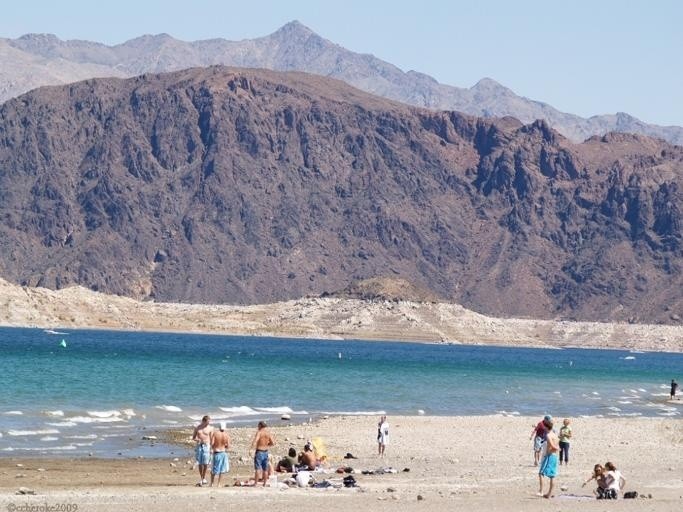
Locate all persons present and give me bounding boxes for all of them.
[274,447,298,472]
[375,415,389,459]
[297,444,316,471]
[668,378,678,399]
[556,419,573,466]
[534,418,561,499]
[250,421,274,488]
[596,461,625,502]
[257,453,274,482]
[529,414,551,467]
[581,463,609,500]
[208,420,231,487]
[307,440,313,452]
[192,415,215,487]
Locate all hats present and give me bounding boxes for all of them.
[545,416,551,420]
[219,422,227,430]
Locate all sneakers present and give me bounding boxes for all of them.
[203,479,207,483]
